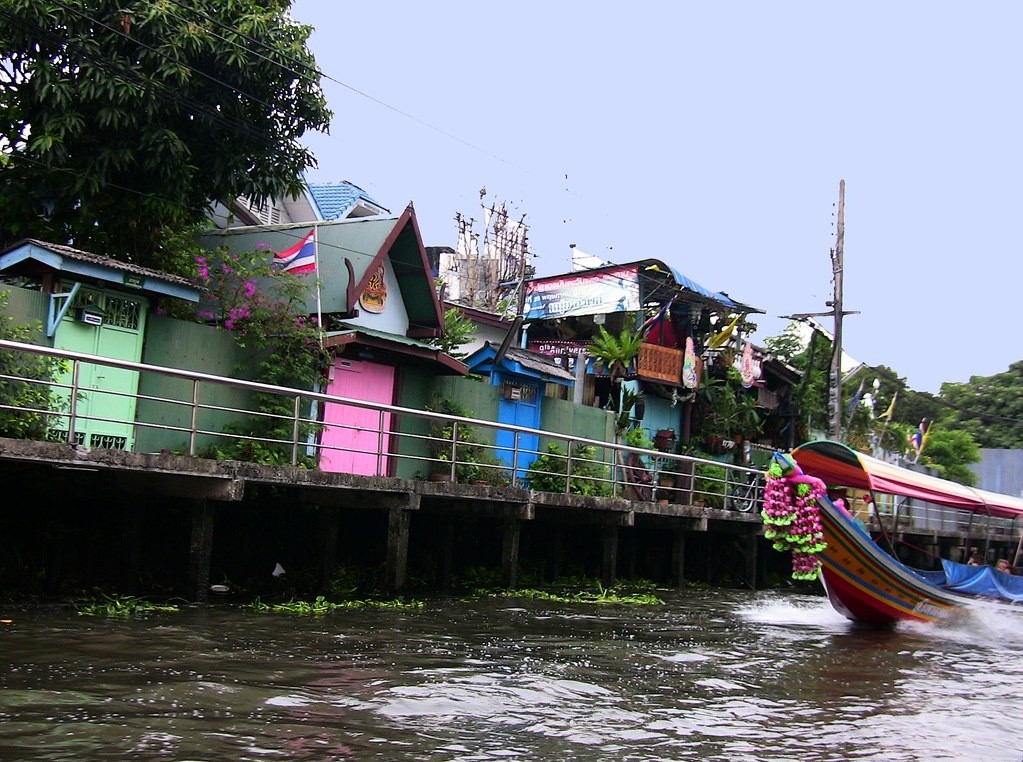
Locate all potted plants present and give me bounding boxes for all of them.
[699,370,766,455]
[473,457,511,487]
[429,426,470,482]
[660,446,694,489]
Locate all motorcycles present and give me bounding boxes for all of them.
[731,463,769,512]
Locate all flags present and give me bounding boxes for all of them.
[637,297,676,348]
[271,231,315,273]
[878,398,895,421]
[848,383,865,408]
[708,317,738,349]
[911,423,925,451]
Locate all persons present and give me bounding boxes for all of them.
[970,556,985,566]
[995,559,1010,574]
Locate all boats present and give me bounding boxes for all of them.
[761,439,1022,649]
